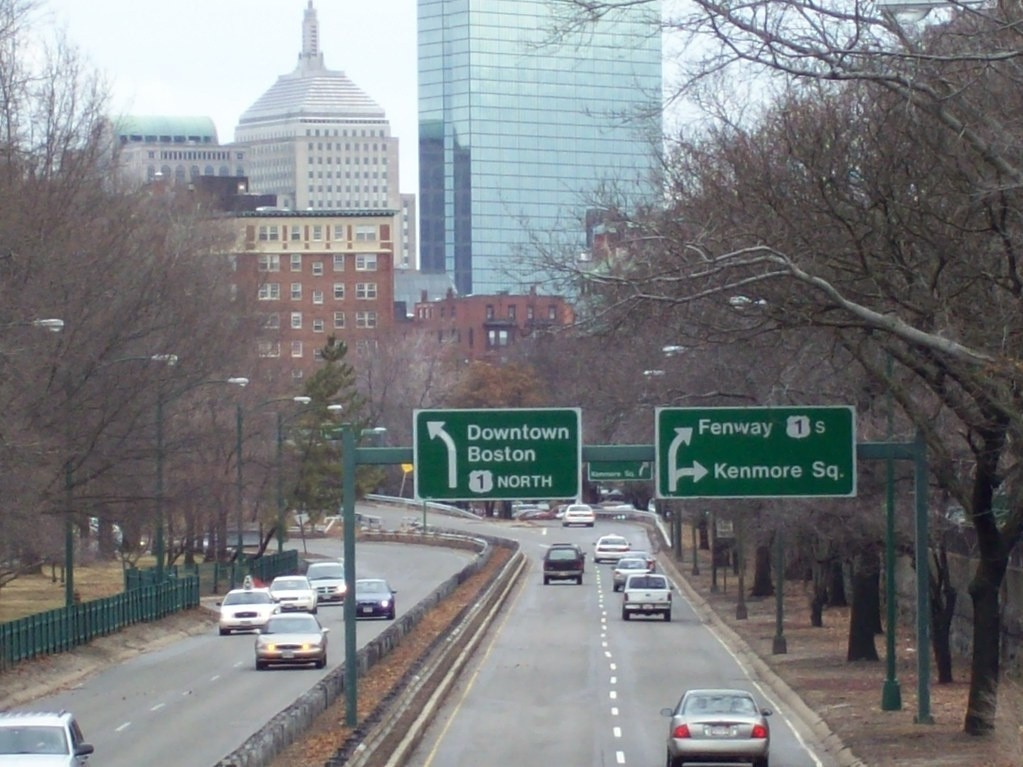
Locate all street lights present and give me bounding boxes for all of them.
[0,317,65,334]
[237,397,313,575]
[276,403,342,551]
[63,353,179,601]
[155,378,249,580]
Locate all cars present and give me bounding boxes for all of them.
[271,576,319,614]
[255,614,330,669]
[306,563,347,602]
[355,579,397,621]
[563,504,595,527]
[593,533,630,563]
[220,588,278,635]
[510,505,559,521]
[612,552,654,590]
[660,689,772,766]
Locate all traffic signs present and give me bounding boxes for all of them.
[412,408,586,502]
[588,459,655,481]
[654,403,858,500]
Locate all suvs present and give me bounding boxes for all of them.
[0,709,92,765]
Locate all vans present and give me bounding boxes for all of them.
[542,542,587,584]
[622,574,673,622]
